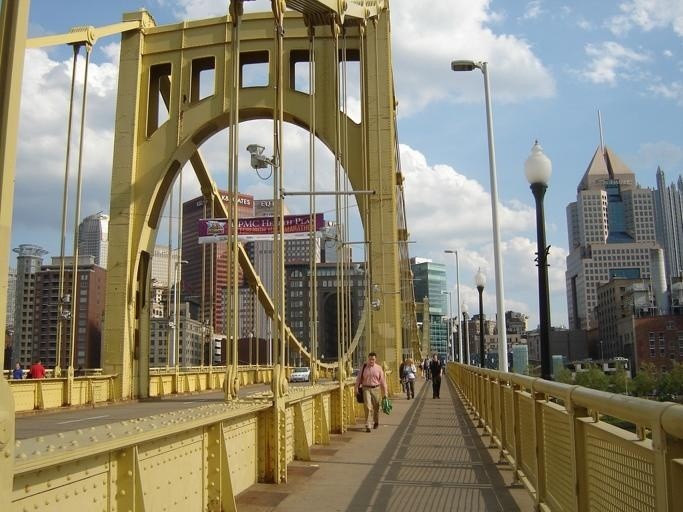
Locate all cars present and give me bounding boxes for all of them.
[336,369,360,381]
[290,367,311,382]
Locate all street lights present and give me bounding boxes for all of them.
[167,260,190,367]
[444,250,463,365]
[461,300,469,364]
[451,58,508,373]
[442,290,457,361]
[475,266,486,369]
[525,139,557,406]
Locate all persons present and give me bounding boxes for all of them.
[11,362,22,380]
[354,351,389,433]
[397,353,444,400]
[26,359,47,380]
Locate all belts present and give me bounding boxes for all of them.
[372,385,379,388]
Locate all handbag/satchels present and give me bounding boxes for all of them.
[403,374,408,384]
[356,387,363,403]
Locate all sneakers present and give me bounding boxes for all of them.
[374,423,378,429]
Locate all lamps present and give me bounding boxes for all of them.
[60,310,72,321]
[245,143,282,183]
[61,292,71,303]
